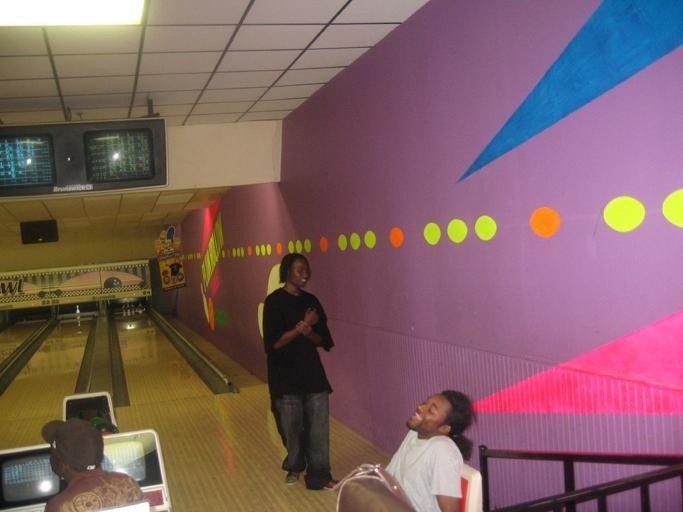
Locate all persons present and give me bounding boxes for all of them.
[40,419,149,512]
[385,390,474,512]
[261,252,340,491]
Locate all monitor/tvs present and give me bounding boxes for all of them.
[99,428,174,486]
[0,120,70,201]
[70,115,170,193]
[0,443,66,510]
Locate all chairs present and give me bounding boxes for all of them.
[459,463,483,512]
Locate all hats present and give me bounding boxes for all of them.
[42,420,104,470]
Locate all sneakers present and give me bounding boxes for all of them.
[322,479,339,491]
[287,469,299,485]
